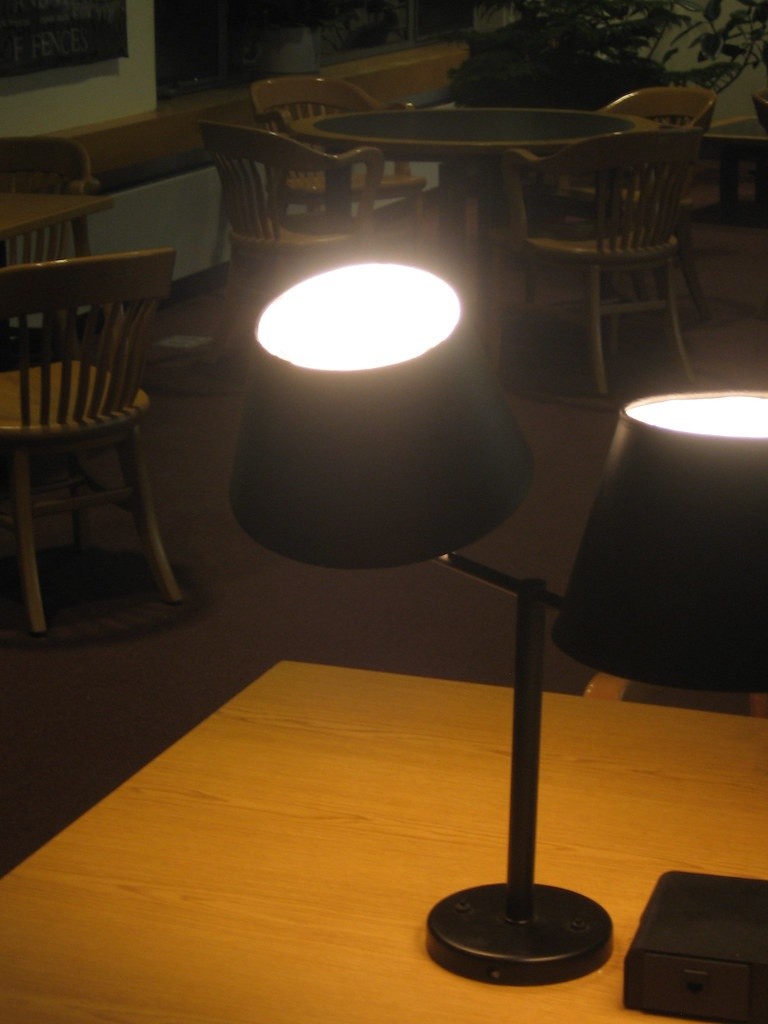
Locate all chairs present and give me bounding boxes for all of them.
[0,136,183,635]
[200,62,720,403]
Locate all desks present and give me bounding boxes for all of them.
[0,191,113,488]
[288,105,658,382]
[699,115,768,224]
[0,661,764,1024]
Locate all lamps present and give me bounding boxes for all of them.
[230,260,767,986]
